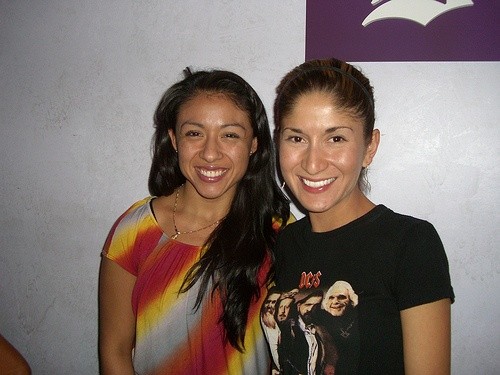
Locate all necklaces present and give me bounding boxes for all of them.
[172,185,229,240]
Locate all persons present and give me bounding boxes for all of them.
[98,66,297,375]
[259,58,454,375]
[0,335,34,375]
